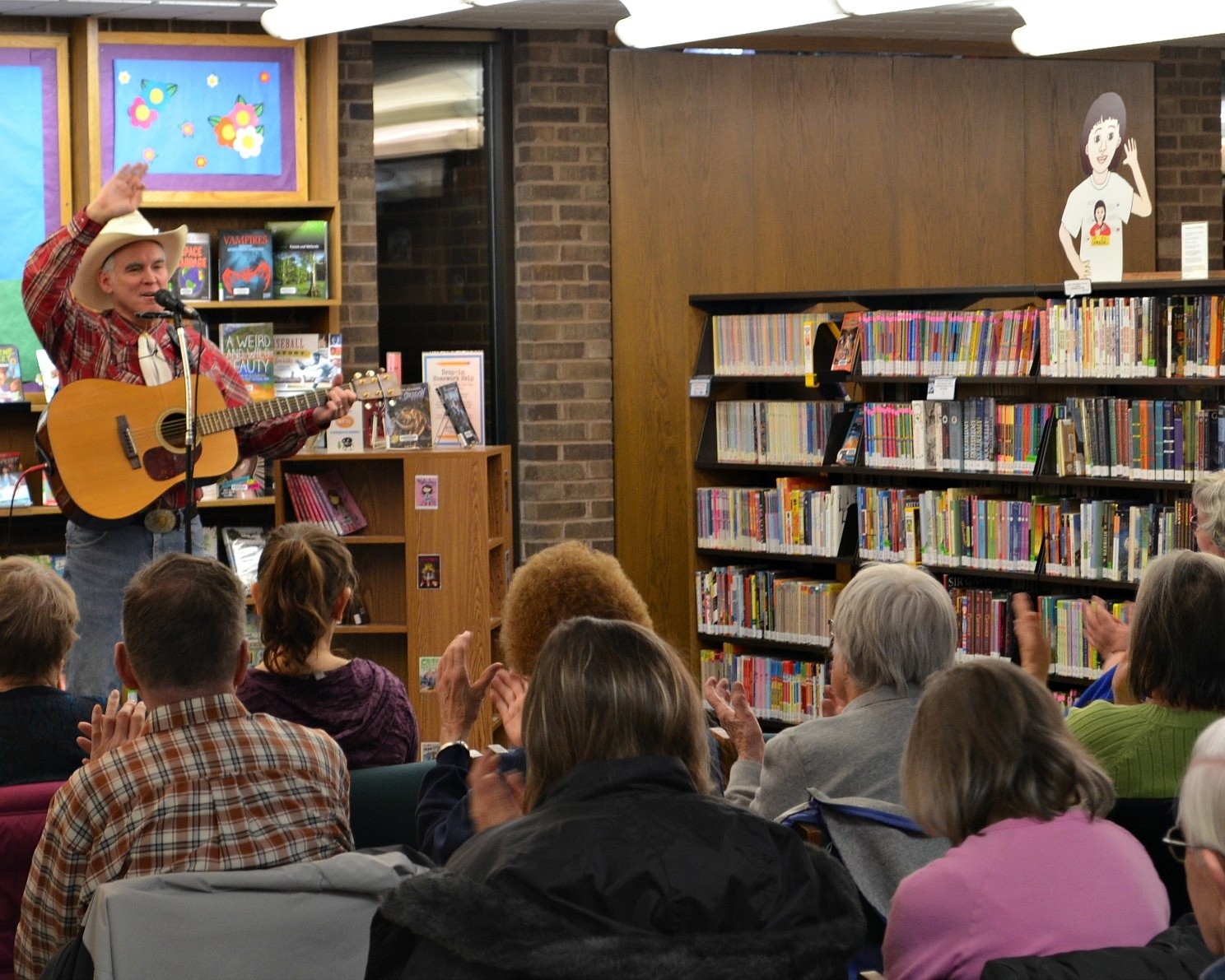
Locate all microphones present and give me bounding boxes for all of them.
[153,289,198,318]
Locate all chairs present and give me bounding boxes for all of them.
[0,774,67,980]
[1105,797,1194,928]
[348,759,436,850]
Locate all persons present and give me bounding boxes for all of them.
[365,475,1225,980]
[22,161,356,692]
[230,522,420,769]
[0,553,113,784]
[9,552,361,979]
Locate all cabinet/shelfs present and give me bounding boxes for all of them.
[688,279,1225,741]
[272,446,515,755]
[0,200,342,607]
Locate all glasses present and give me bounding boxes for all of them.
[826,618,837,635]
[1164,825,1224,862]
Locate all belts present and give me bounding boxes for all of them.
[133,500,197,533]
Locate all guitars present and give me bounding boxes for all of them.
[35,368,404,532]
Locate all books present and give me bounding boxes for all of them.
[695,295,1225,724]
[217,226,273,302]
[164,231,210,306]
[0,452,31,509]
[28,553,66,577]
[41,469,60,507]
[35,349,59,405]
[220,524,268,594]
[267,219,329,300]
[0,344,24,404]
[219,454,266,499]
[281,467,368,538]
[200,526,219,563]
[198,321,340,404]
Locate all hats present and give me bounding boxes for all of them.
[69,209,188,312]
